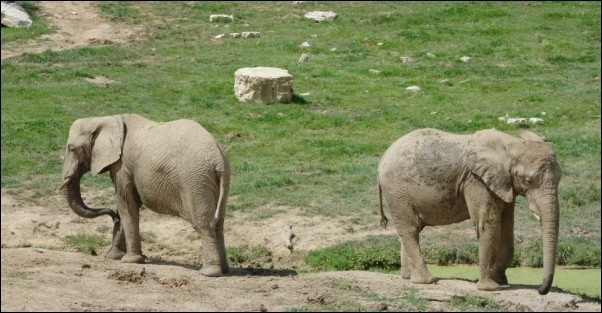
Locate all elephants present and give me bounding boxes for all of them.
[57,112,231,276]
[377,126,563,295]
